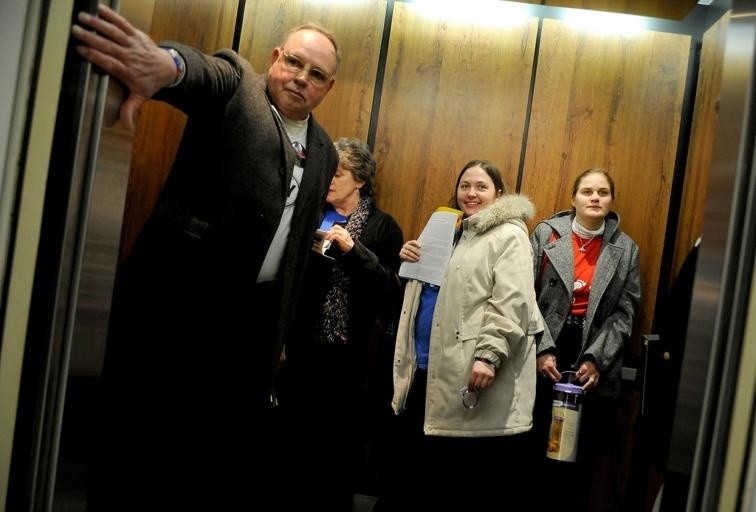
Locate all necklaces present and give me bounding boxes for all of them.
[578,227,596,253]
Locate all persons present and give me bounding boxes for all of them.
[528,168,641,510]
[388,158,545,511]
[71,3,340,511]
[292,137,404,512]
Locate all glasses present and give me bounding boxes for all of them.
[281,45,334,90]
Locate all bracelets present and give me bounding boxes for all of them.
[167,49,183,84]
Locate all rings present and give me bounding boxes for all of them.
[579,369,584,375]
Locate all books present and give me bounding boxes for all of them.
[399,207,464,287]
